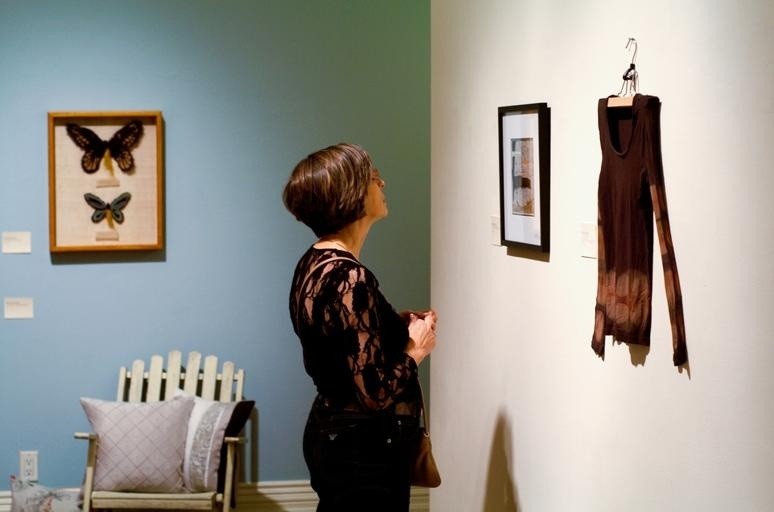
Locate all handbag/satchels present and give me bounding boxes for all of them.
[409,375,442,488]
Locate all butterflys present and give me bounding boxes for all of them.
[66,120,144,178]
[84,192,131,230]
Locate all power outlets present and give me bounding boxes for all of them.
[18,452,39,484]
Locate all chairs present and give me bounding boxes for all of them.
[73,348,248,511]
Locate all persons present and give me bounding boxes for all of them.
[281,140,442,512]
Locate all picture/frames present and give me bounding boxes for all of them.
[48,111,165,253]
[498,102,550,254]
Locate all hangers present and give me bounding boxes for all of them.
[607,37,634,109]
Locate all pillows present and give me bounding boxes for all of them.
[10,475,83,512]
[81,397,195,492]
[175,389,255,492]
